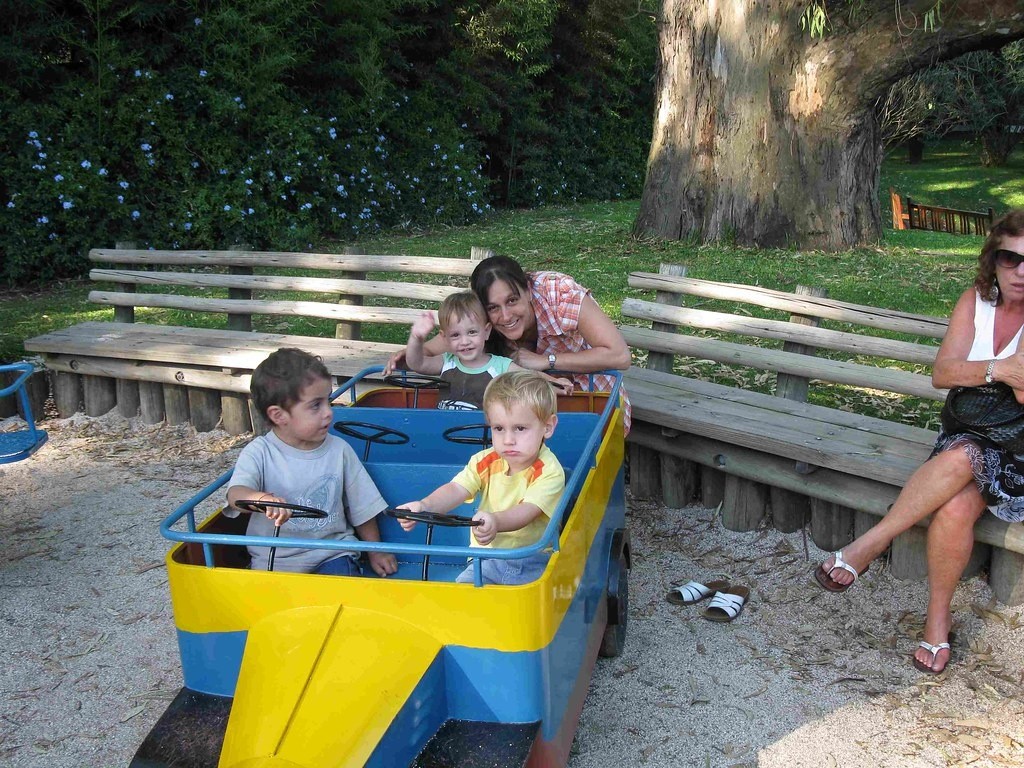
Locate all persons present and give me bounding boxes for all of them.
[395,370,566,585]
[382,256,632,438]
[406,293,573,411]
[223,347,398,578]
[813,208,1024,674]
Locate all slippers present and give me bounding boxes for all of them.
[665,579,731,606]
[702,584,750,622]
[814,549,869,592]
[913,632,956,676]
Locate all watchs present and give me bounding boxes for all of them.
[549,353,556,369]
[986,361,996,385]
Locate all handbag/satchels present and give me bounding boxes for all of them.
[941,381,1024,455]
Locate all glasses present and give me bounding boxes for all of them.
[992,248,1024,268]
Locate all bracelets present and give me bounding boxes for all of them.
[258,493,274,500]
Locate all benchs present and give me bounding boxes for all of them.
[618,264,1024,607]
[323,389,616,589]
[23,242,496,431]
